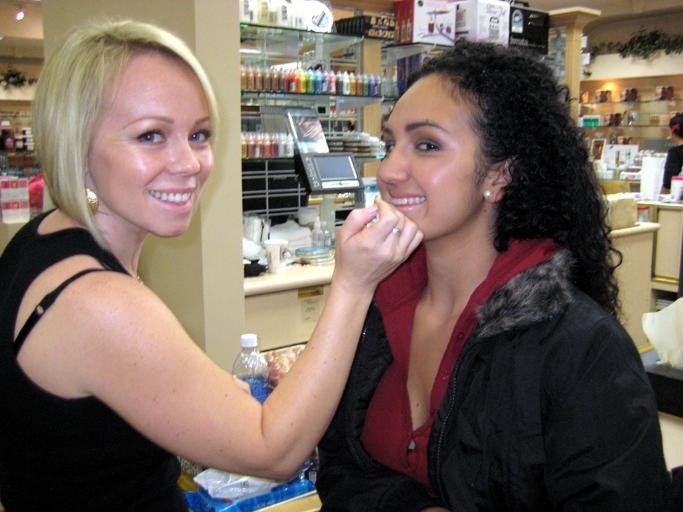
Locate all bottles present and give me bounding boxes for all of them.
[241,131,294,159]
[241,64,382,96]
[311,216,332,250]
[232,333,270,404]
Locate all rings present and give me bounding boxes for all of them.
[393,228,402,236]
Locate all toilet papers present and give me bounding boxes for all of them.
[639,157,666,200]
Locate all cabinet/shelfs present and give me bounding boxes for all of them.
[241,22,399,159]
[580,98,683,127]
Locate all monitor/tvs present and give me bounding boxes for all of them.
[300,150,364,196]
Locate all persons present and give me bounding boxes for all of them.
[316,42,668,512]
[0,20,425,512]
[660,112,683,194]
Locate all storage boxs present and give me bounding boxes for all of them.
[455,0,511,49]
[393,0,458,46]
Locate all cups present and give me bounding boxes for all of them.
[263,240,290,274]
[670,176,683,204]
